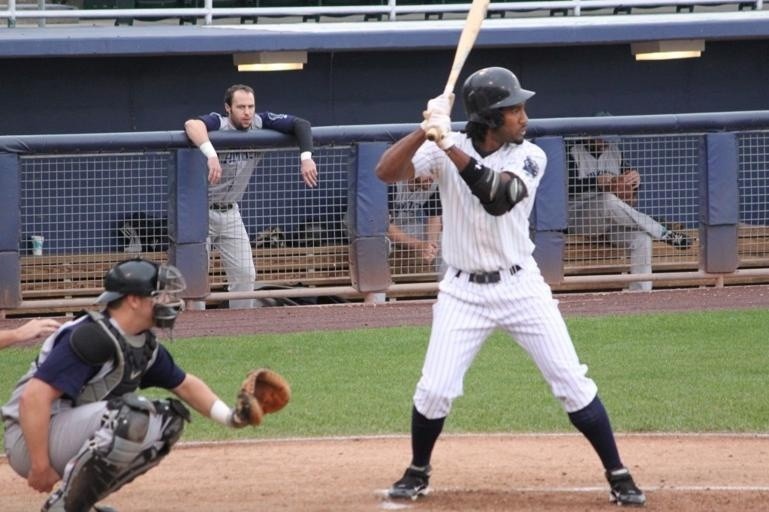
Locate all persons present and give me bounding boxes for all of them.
[0,254,253,511]
[565,108,696,292]
[183,82,320,310]
[372,63,651,507]
[0,317,65,349]
[343,162,443,303]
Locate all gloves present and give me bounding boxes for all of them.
[422,109,455,150]
[420,94,450,133]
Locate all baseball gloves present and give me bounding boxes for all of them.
[610,170,638,206]
[231,370,289,429]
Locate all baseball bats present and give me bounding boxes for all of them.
[425,0,491,139]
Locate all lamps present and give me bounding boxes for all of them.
[230,49,309,74]
[627,41,708,63]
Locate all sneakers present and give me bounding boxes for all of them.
[662,229,693,250]
[605,473,646,504]
[389,467,431,500]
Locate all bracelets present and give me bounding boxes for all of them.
[201,142,217,159]
[439,136,456,148]
[208,396,233,427]
[299,150,313,160]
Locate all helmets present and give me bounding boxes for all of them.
[93,258,187,341]
[462,66,536,127]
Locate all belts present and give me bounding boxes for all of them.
[456,264,522,285]
[210,204,232,212]
